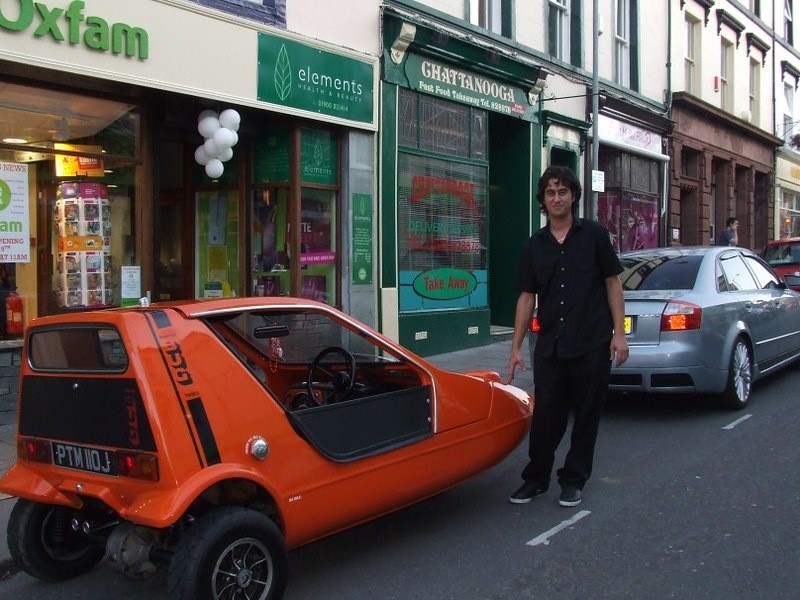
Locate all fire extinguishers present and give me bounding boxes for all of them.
[5,287,24,336]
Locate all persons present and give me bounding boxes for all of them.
[257,286,265,296]
[720,217,739,247]
[504,164,630,508]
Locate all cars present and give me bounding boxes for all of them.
[0,295,535,600]
[761,237,799,294]
[529,245,800,409]
[398,237,487,273]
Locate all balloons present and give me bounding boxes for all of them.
[194,109,241,178]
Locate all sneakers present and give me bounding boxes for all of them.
[556,475,584,507]
[509,477,554,504]
